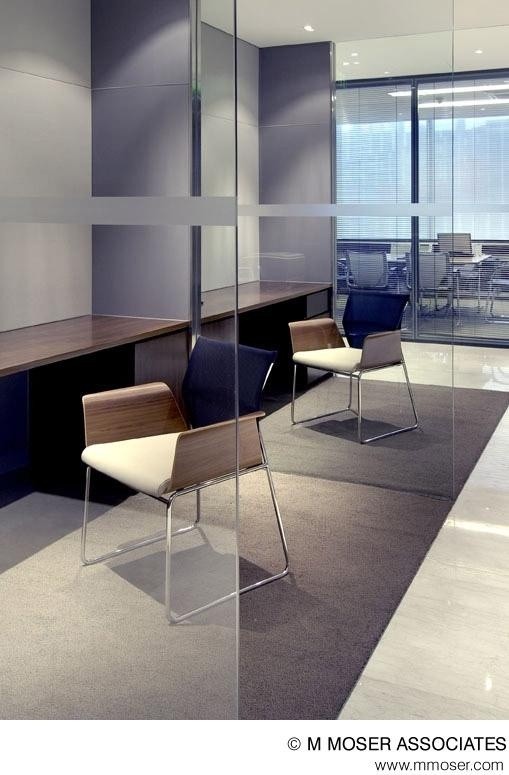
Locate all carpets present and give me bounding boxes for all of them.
[1,374,508,720]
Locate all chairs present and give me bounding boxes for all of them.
[336,231,508,329]
[285,287,421,448]
[78,334,293,627]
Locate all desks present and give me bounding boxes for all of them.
[237,277,335,394]
[0,314,194,509]
